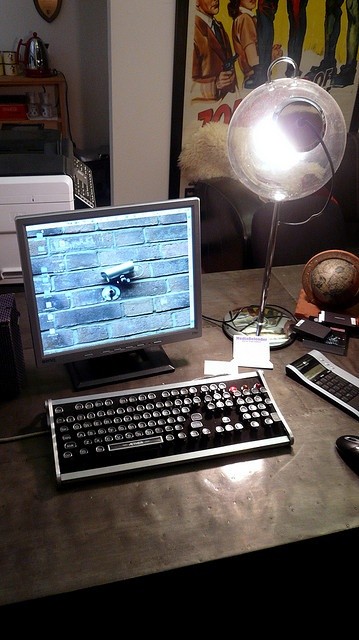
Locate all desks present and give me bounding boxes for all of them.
[0,264,359,606]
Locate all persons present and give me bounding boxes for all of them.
[245,0,308,88]
[303,0,359,88]
[190,0,236,106]
[227,0,263,88]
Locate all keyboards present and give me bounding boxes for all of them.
[42,370,288,483]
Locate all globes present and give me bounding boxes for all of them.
[302,250,359,316]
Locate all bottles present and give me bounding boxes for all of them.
[40,93,53,118]
[27,90,40,117]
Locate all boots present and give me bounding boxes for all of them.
[335,64,357,87]
[304,58,336,83]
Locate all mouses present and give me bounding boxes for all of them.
[335,435,359,479]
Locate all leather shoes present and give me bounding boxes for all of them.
[244,66,272,88]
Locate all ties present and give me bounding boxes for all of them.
[212,19,226,53]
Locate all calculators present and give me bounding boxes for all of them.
[284,348,359,421]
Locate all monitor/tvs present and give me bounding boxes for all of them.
[15,197,202,392]
[0,175,75,278]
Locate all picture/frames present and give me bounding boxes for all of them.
[167,3,359,273]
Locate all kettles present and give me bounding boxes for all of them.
[15,31,52,78]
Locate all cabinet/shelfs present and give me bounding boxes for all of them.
[1,70,66,140]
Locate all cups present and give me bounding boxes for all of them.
[4,63,19,77]
[4,50,17,64]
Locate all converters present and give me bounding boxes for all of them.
[293,318,331,341]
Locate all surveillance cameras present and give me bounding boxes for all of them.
[100,260,135,285]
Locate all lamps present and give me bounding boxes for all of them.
[223,56,347,349]
